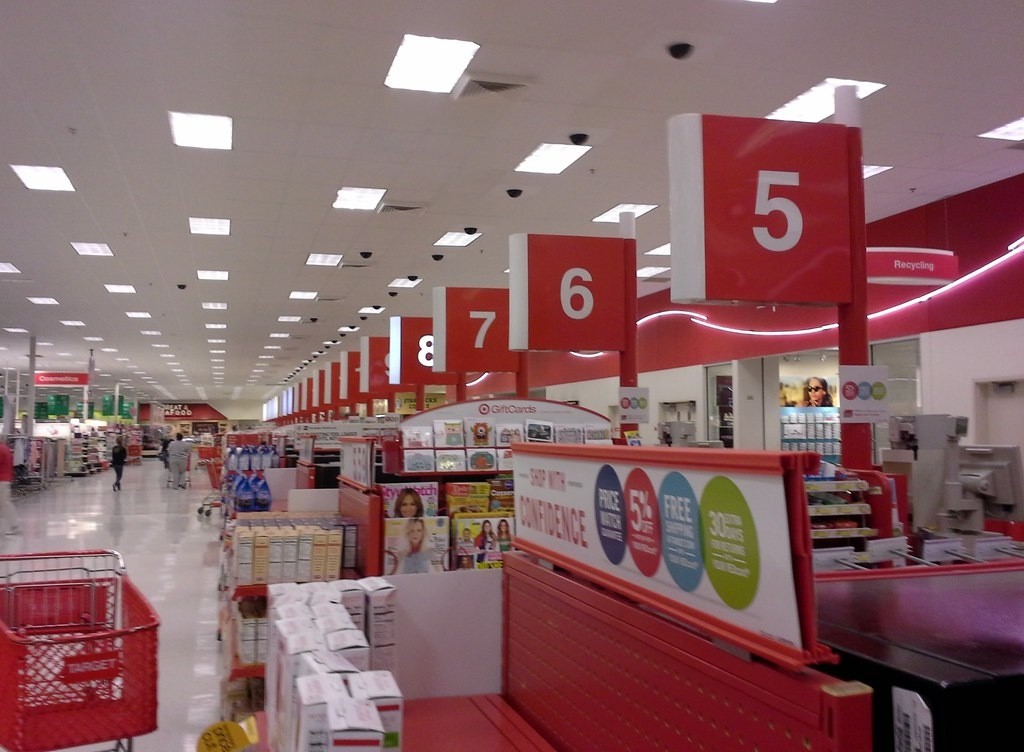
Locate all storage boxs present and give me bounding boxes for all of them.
[229,512,402,752]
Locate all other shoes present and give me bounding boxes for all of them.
[6,525,24,534]
[112,484,116,491]
[118,486,120,490]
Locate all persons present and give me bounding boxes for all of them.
[398,518,444,574]
[458,520,512,569]
[393,488,424,519]
[161,439,174,469]
[167,433,190,491]
[796,377,834,407]
[111,437,127,492]
[0,434,22,535]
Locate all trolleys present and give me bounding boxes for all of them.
[195,458,222,516]
[0,547,161,752]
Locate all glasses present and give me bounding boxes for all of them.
[808,386,823,392]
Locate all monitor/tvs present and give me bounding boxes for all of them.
[959,445,1024,522]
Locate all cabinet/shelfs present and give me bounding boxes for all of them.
[804,469,892,568]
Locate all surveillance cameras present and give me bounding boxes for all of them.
[464,228,478,234]
[570,134,590,146]
[669,44,695,59]
[507,189,522,198]
[283,252,443,384]
[177,285,186,290]
